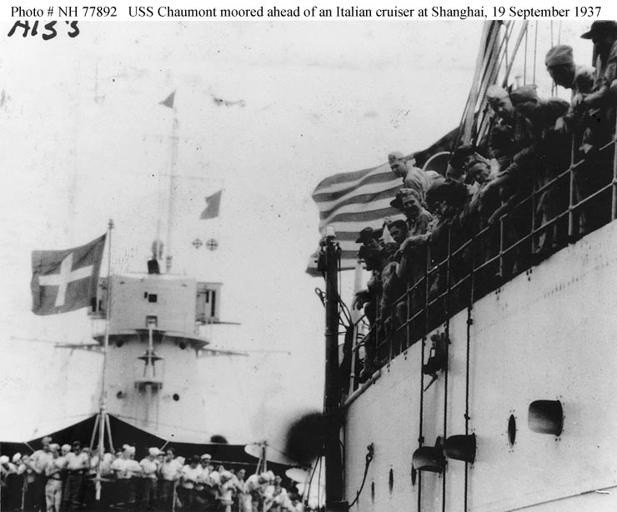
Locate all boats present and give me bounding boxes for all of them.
[342,17,617,510]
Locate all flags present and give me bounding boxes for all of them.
[200,189,222,219]
[31,231,107,315]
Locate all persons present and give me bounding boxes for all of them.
[2,441,308,511]
[355,21,615,383]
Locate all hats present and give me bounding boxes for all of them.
[2,436,312,482]
[355,19,616,246]
